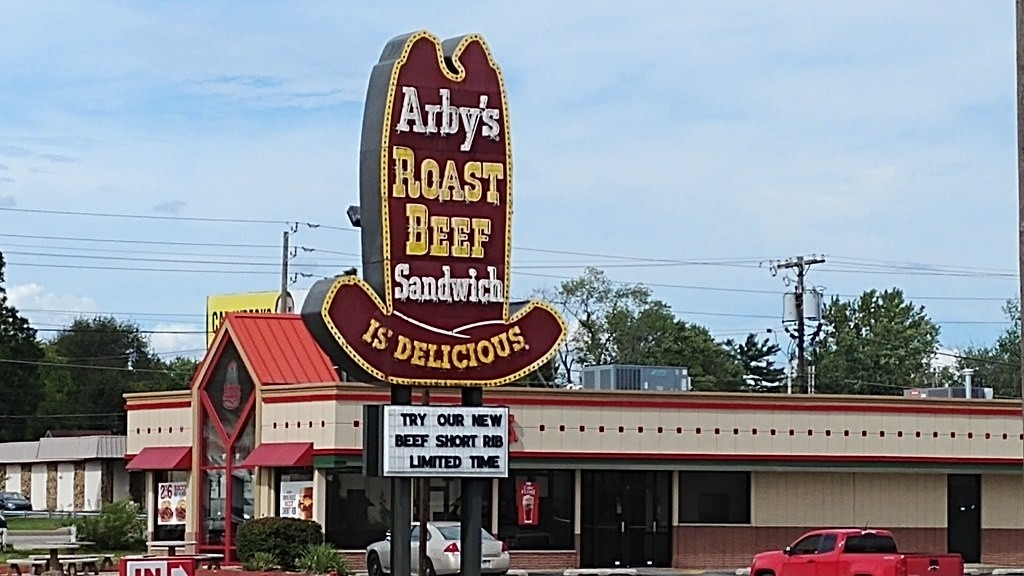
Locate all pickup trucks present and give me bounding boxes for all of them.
[748,528,965,575]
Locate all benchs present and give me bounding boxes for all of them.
[189,554,224,569]
[6,555,115,576]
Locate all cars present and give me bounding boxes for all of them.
[0,492,33,510]
[364,521,511,576]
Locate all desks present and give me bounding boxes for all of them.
[33,540,80,576]
[146,540,198,556]
[46,541,96,576]
